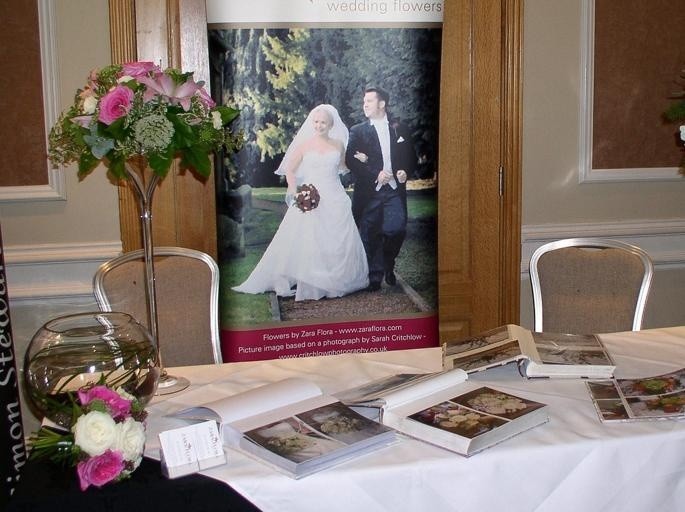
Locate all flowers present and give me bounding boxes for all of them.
[45,55,247,180]
[31,386,152,490]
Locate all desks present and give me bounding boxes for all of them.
[527,233,654,340]
[16,326,684,512]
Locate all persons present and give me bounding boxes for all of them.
[230,104,370,302]
[345,85,418,292]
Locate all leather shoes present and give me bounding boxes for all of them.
[369,272,396,290]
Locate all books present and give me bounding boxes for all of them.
[331,369,549,458]
[441,324,617,382]
[163,376,401,480]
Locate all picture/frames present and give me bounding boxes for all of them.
[576,2,685,185]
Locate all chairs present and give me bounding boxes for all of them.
[91,243,225,373]
